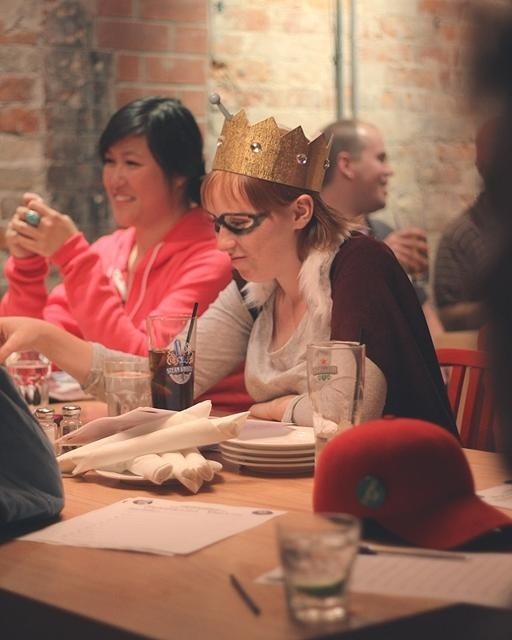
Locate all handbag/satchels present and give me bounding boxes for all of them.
[0,366,67,544]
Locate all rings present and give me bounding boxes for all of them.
[23,209,42,226]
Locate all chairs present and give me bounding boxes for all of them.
[434,348,504,453]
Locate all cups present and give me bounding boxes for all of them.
[273,512,363,623]
[8,357,54,410]
[303,340,366,468]
[102,356,152,417]
[391,203,429,286]
[145,313,197,413]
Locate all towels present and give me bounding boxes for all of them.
[56,399,251,494]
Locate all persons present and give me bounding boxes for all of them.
[433,114,510,348]
[317,116,429,307]
[0,92,256,416]
[1,167,464,448]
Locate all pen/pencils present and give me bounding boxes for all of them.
[229,573,260,616]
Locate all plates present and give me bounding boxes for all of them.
[93,461,224,487]
[219,423,315,476]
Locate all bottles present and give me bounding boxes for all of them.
[35,405,59,457]
[59,406,85,455]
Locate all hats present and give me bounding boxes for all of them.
[309,414,512,552]
